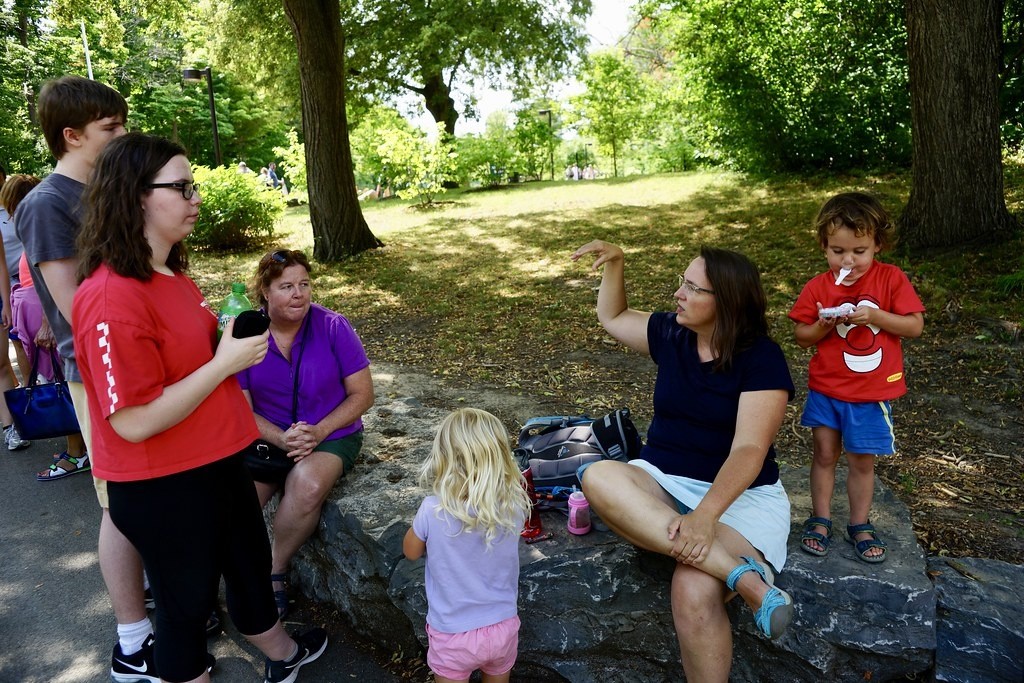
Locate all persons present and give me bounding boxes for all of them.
[786,193,926,562]
[237,160,281,188]
[565,163,596,180]
[403,408,533,683]
[15,74,224,640]
[572,239,795,683]
[0,166,89,481]
[237,248,373,620]
[71,134,329,683]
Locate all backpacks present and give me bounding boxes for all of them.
[519,406,646,507]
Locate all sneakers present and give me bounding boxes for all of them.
[264,627,328,683]
[110,634,215,683]
[144,586,158,612]
[3,427,31,450]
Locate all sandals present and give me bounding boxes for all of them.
[271,571,290,620]
[727,554,794,641]
[844,518,888,562]
[799,516,833,557]
[36,450,92,482]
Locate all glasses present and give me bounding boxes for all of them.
[678,275,715,296]
[147,181,201,199]
[259,250,306,277]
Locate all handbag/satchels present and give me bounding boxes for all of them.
[244,436,294,485]
[3,344,81,441]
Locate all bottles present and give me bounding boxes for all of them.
[217,282,254,348]
[510,448,542,537]
[567,484,592,535]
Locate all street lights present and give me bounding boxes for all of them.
[538,109,555,180]
[584,142,593,168]
[184,66,222,167]
